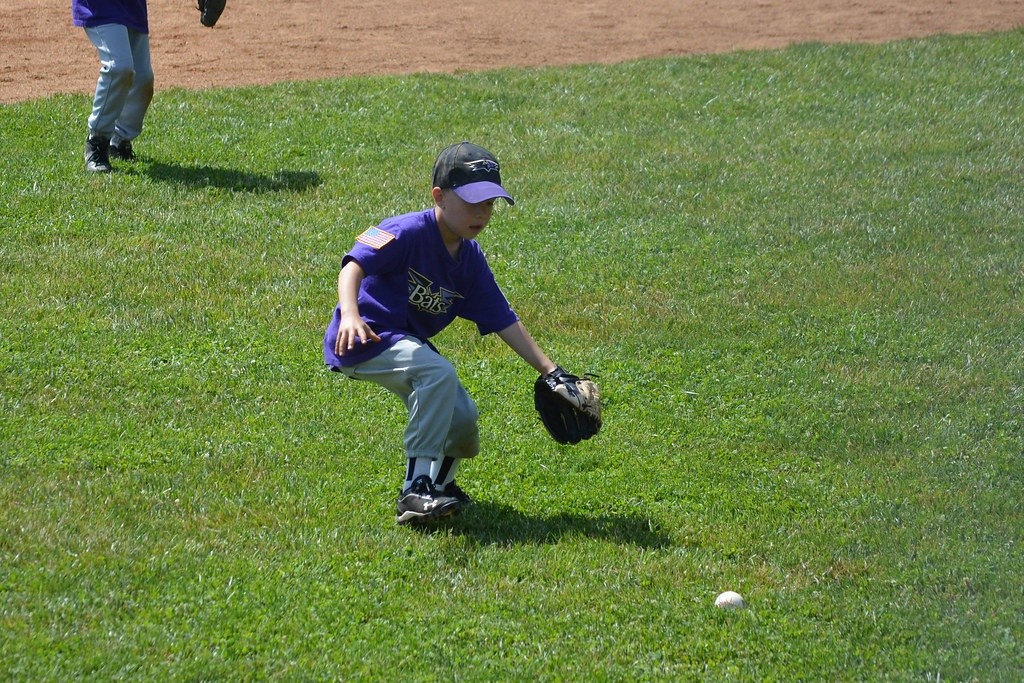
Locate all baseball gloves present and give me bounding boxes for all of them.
[534,366,605,445]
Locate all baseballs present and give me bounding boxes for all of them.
[715,589,747,611]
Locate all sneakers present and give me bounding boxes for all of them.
[394,474,459,524]
[84,134,111,173]
[108,140,136,163]
[434,478,477,507]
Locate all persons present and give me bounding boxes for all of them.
[72,0,227,170]
[324,142,603,523]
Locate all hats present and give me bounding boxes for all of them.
[432,141,515,206]
